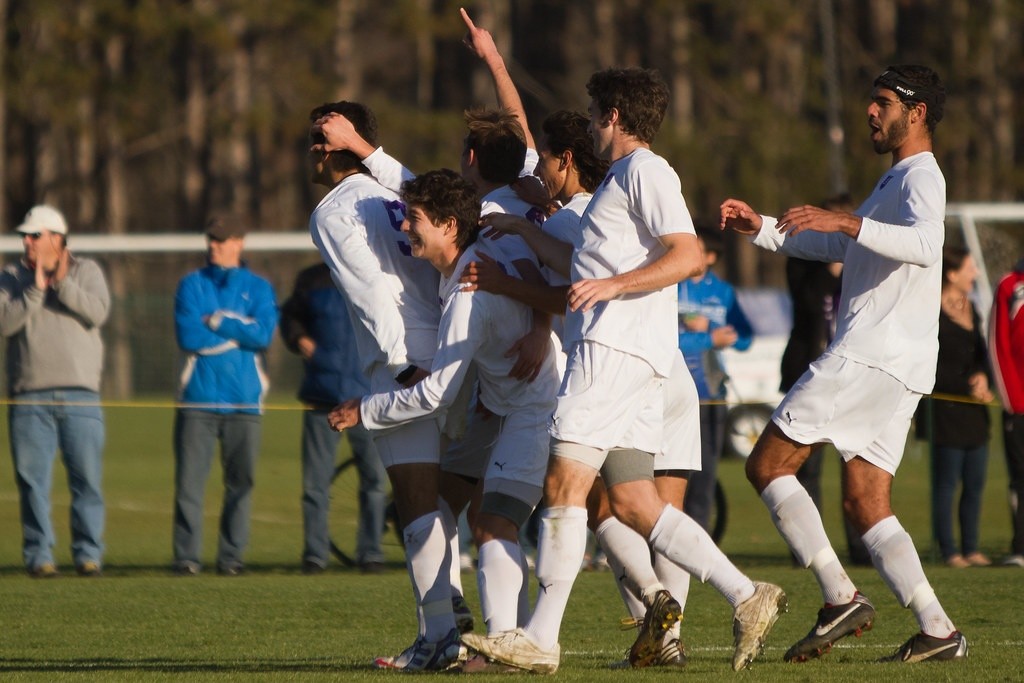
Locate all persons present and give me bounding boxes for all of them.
[779,192,878,572]
[165,210,280,577]
[1,204,113,579]
[274,260,388,576]
[677,215,758,534]
[305,6,788,670]
[927,243,998,570]
[720,65,968,664]
[988,259,1024,567]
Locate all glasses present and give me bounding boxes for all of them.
[19,232,41,240]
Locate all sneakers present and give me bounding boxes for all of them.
[373,627,467,672]
[731,582,789,672]
[462,627,560,674]
[659,639,687,666]
[629,590,684,669]
[463,652,521,673]
[784,591,876,663]
[876,629,969,665]
[450,596,475,633]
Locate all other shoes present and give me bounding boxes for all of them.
[81,563,101,576]
[36,565,59,577]
[303,561,319,574]
[1005,555,1024,567]
[219,566,236,576]
[362,563,385,574]
[179,566,195,575]
[965,554,993,567]
[946,556,969,569]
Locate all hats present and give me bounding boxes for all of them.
[205,213,247,241]
[15,205,68,238]
